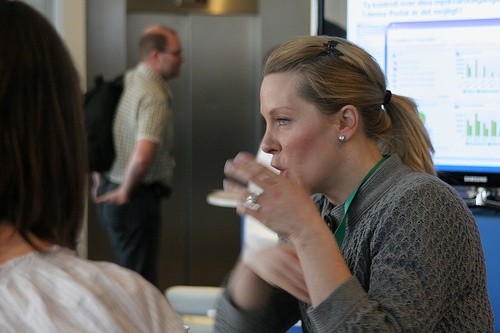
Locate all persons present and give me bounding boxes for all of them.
[213,35,495,333]
[92,25,185,287]
[0,0,190,333]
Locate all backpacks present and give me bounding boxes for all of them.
[83,71,125,177]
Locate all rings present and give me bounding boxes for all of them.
[245,192,256,204]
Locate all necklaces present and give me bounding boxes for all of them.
[319,153,390,248]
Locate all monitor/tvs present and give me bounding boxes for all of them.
[309,0,500,212]
